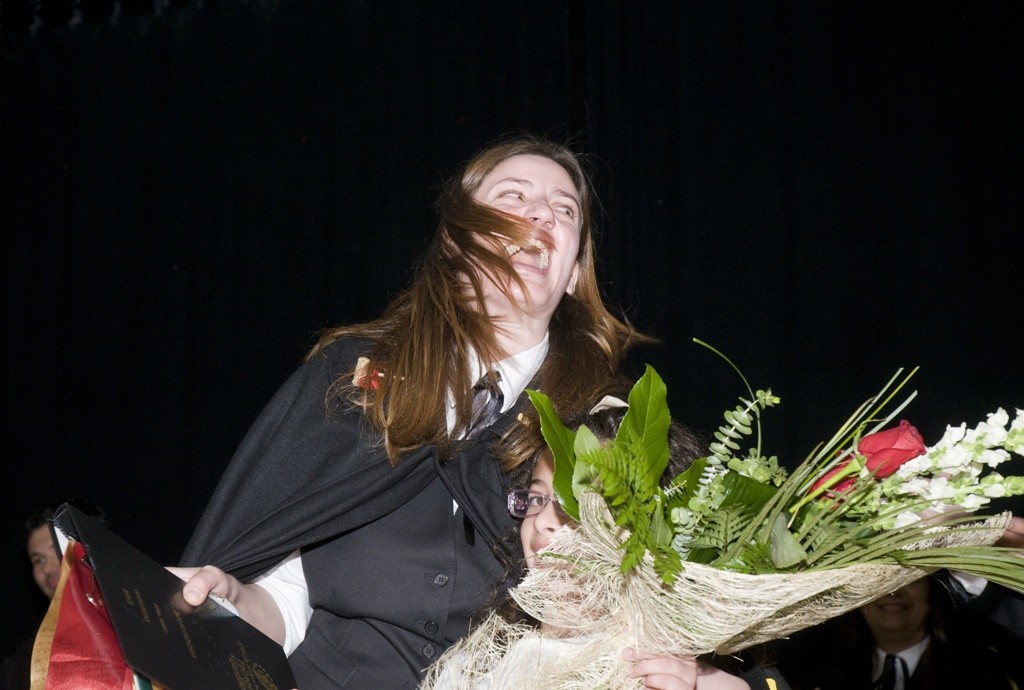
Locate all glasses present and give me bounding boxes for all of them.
[507,489,574,517]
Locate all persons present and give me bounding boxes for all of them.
[163,133,633,690]
[814,576,1013,690]
[22,500,106,603]
[493,409,749,689]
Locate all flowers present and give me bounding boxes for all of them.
[415,339,1024,690]
